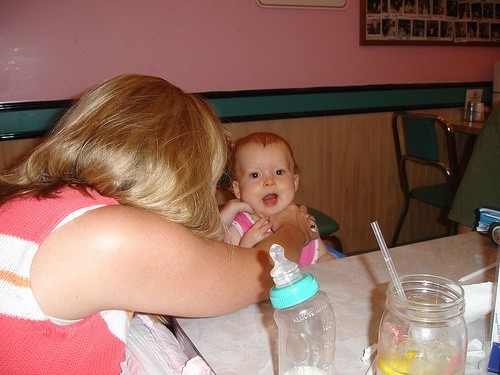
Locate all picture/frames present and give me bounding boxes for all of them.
[359,0,500,47]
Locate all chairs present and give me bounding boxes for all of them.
[388,110,459,247]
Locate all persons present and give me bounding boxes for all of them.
[367,0,500,42]
[224,131,336,268]
[0,72,320,375]
[448,97,500,237]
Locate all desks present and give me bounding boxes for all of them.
[451,114,486,134]
[174,233,500,375]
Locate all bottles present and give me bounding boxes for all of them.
[379,274,468,374]
[269,243,337,375]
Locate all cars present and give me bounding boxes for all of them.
[475,206,500,247]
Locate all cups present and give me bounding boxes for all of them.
[467,101,491,113]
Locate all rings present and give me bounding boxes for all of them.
[307,216,318,232]
